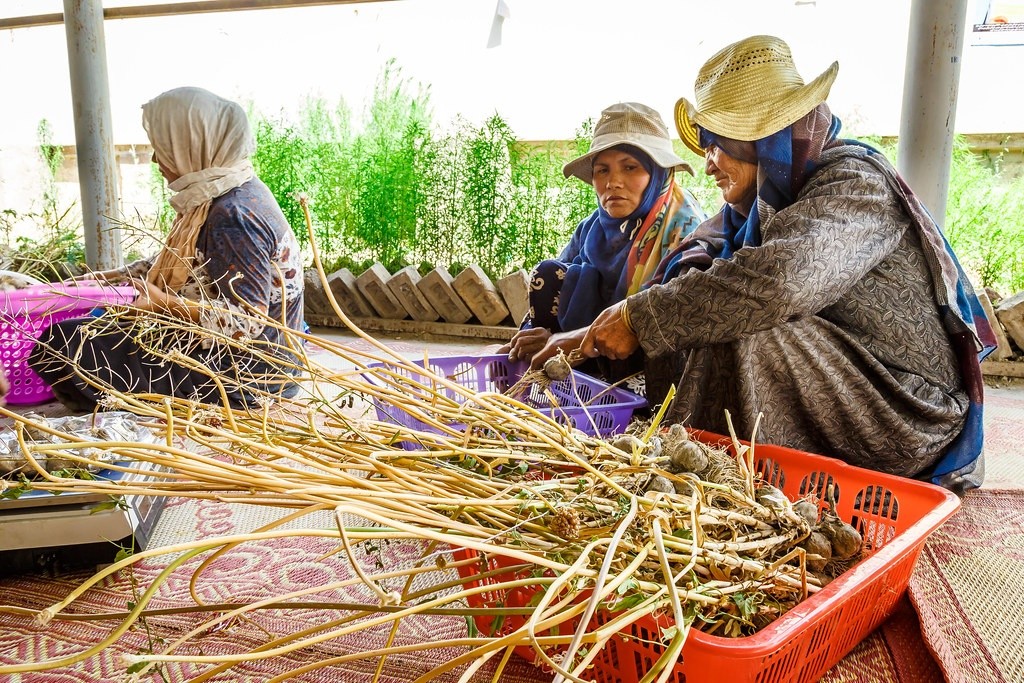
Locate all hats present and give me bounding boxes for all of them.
[676,35,839,158]
[563,102,694,182]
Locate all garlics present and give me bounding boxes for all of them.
[0,411,138,475]
[535,355,863,592]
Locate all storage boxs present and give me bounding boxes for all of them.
[357,356,647,469]
[450,426,963,683]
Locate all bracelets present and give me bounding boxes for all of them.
[621,301,637,337]
[84,272,92,279]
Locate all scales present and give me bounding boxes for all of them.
[0,433,178,574]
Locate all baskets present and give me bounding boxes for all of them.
[0,280,141,404]
[355,354,649,474]
[451,425,962,683]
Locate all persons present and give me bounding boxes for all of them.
[530,36,999,518]
[491,103,708,420]
[27,88,304,411]
